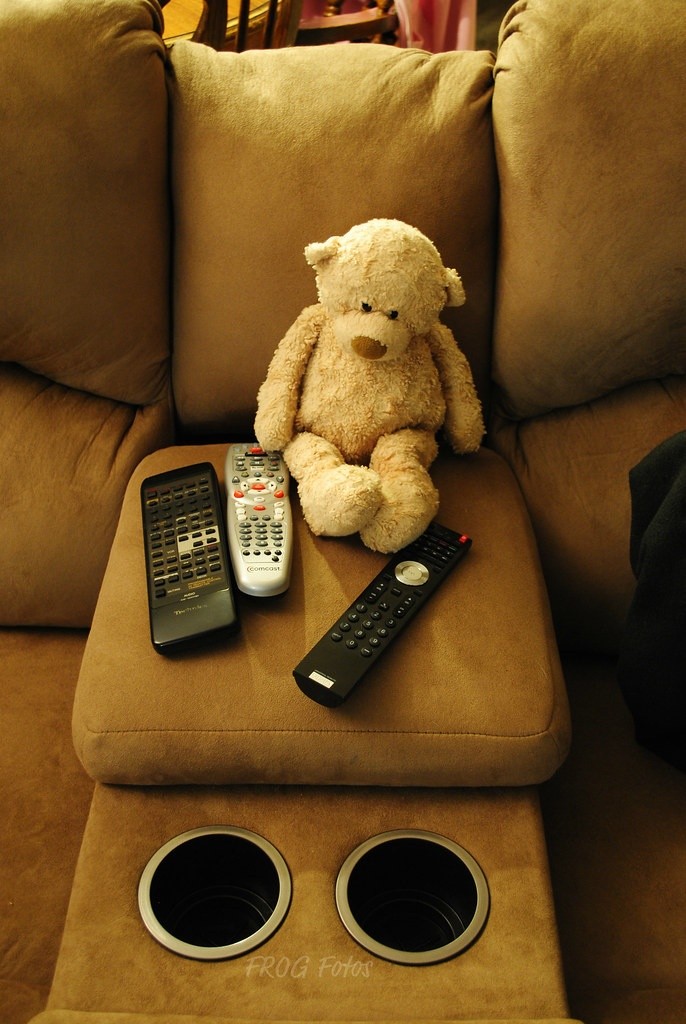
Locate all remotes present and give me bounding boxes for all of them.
[140,462,239,655]
[225,443,293,597]
[293,520,472,707]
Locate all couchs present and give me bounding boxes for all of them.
[0,1,686,1024]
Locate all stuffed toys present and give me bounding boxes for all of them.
[254,219,484,553]
